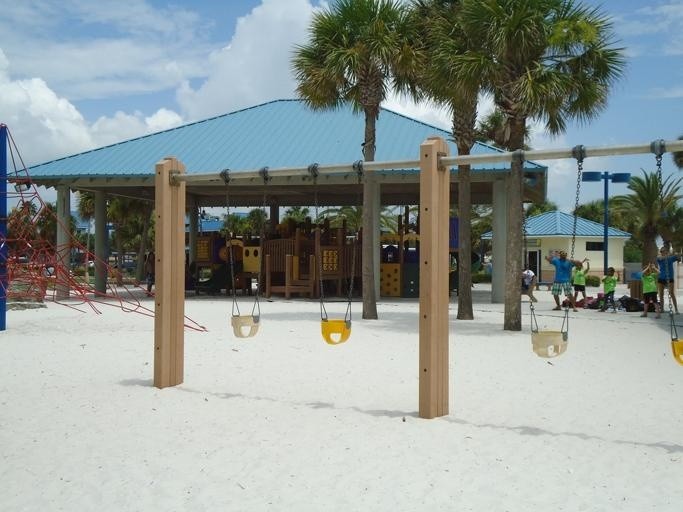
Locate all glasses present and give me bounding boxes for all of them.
[660,250,665,253]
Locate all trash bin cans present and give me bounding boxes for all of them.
[628,280,641,299]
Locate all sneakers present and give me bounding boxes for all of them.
[614,307,616,312]
[598,309,605,312]
[640,313,647,317]
[584,304,588,308]
[655,315,661,319]
[675,312,679,314]
[573,308,578,311]
[553,306,561,310]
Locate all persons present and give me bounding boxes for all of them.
[145,252,154,296]
[569,260,590,309]
[522,264,535,290]
[655,246,682,314]
[596,267,618,314]
[639,262,662,319]
[544,250,589,312]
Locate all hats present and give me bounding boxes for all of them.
[559,250,567,258]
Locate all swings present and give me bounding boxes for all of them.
[224,178,267,337]
[518,257,519,261]
[656,155,683,366]
[313,173,363,345]
[517,159,583,358]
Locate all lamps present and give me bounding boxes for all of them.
[15,182,31,192]
